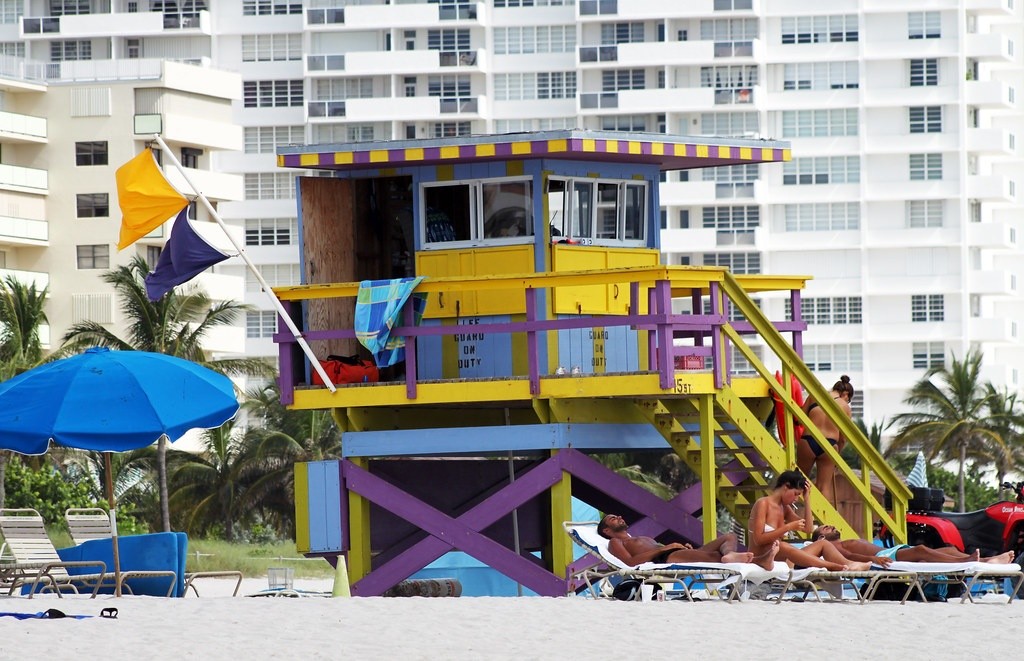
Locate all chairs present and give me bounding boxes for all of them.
[562,520,1024,604]
[0,507,70,578]
[65,507,113,546]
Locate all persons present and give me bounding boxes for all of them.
[748,467,872,572]
[812,525,1016,569]
[597,514,780,572]
[796,374,853,502]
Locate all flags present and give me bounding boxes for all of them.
[144,205,232,304]
[114,147,190,255]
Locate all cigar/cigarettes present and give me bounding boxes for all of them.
[791,501,799,510]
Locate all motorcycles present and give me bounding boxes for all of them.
[873,482,1024,601]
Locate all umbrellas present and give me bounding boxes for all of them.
[0,346,241,598]
